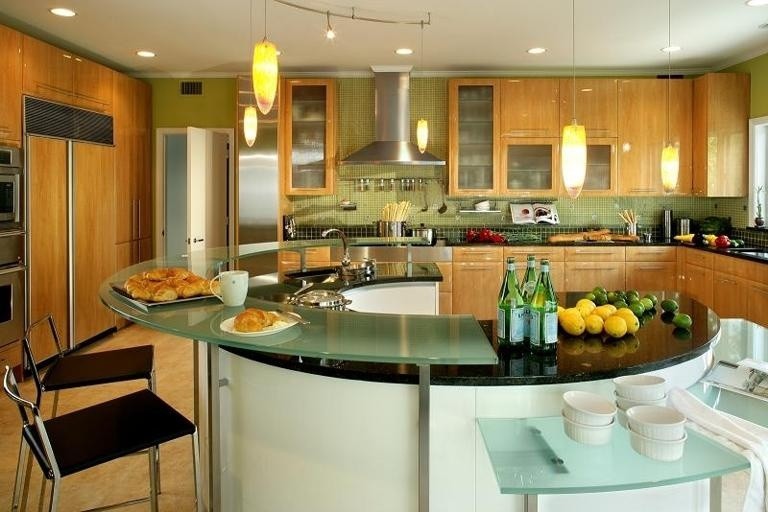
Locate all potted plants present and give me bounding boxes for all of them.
[754,186,764,226]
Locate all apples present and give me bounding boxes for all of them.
[714,235,731,249]
[466,228,507,243]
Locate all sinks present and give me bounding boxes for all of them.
[283,269,338,284]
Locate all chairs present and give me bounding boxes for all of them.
[2,360,205,512]
[17,310,163,512]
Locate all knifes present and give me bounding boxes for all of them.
[274,306,311,327]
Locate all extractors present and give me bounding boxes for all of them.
[337,66,449,166]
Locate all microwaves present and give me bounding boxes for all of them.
[0,147,21,226]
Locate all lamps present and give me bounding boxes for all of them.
[414,19,430,155]
[324,10,335,39]
[556,1,590,200]
[240,73,259,147]
[249,1,282,117]
[657,1,683,192]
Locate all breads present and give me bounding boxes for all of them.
[125,266,220,302]
[548,229,639,242]
[234,308,291,333]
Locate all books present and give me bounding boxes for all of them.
[700,356,768,404]
[510,202,560,225]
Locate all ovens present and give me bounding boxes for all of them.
[0,227,26,349]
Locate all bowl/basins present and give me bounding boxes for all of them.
[563,374,689,461]
[473,199,490,211]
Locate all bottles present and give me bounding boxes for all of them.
[496,354,558,378]
[496,253,558,351]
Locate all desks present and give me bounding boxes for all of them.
[473,318,768,512]
[96,234,500,512]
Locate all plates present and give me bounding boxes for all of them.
[233,325,303,346]
[219,310,303,338]
[108,280,219,306]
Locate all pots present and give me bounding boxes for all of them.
[297,290,350,312]
[373,220,407,246]
[410,222,437,247]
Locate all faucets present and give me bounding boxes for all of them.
[322,227,350,265]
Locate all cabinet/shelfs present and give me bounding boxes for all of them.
[678,244,714,309]
[503,245,565,295]
[447,76,500,198]
[715,253,768,329]
[617,78,692,197]
[279,75,338,196]
[23,36,113,112]
[112,236,152,331]
[566,244,625,293]
[500,78,616,199]
[690,72,748,198]
[278,245,330,285]
[115,70,153,245]
[451,248,502,321]
[0,23,23,146]
[625,245,677,293]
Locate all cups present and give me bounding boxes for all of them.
[624,222,638,236]
[210,269,249,308]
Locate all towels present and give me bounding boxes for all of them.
[668,377,768,512]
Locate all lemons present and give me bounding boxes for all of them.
[672,328,692,341]
[661,311,675,324]
[730,239,745,248]
[556,287,658,338]
[661,299,679,312]
[671,314,694,329]
[560,334,640,358]
